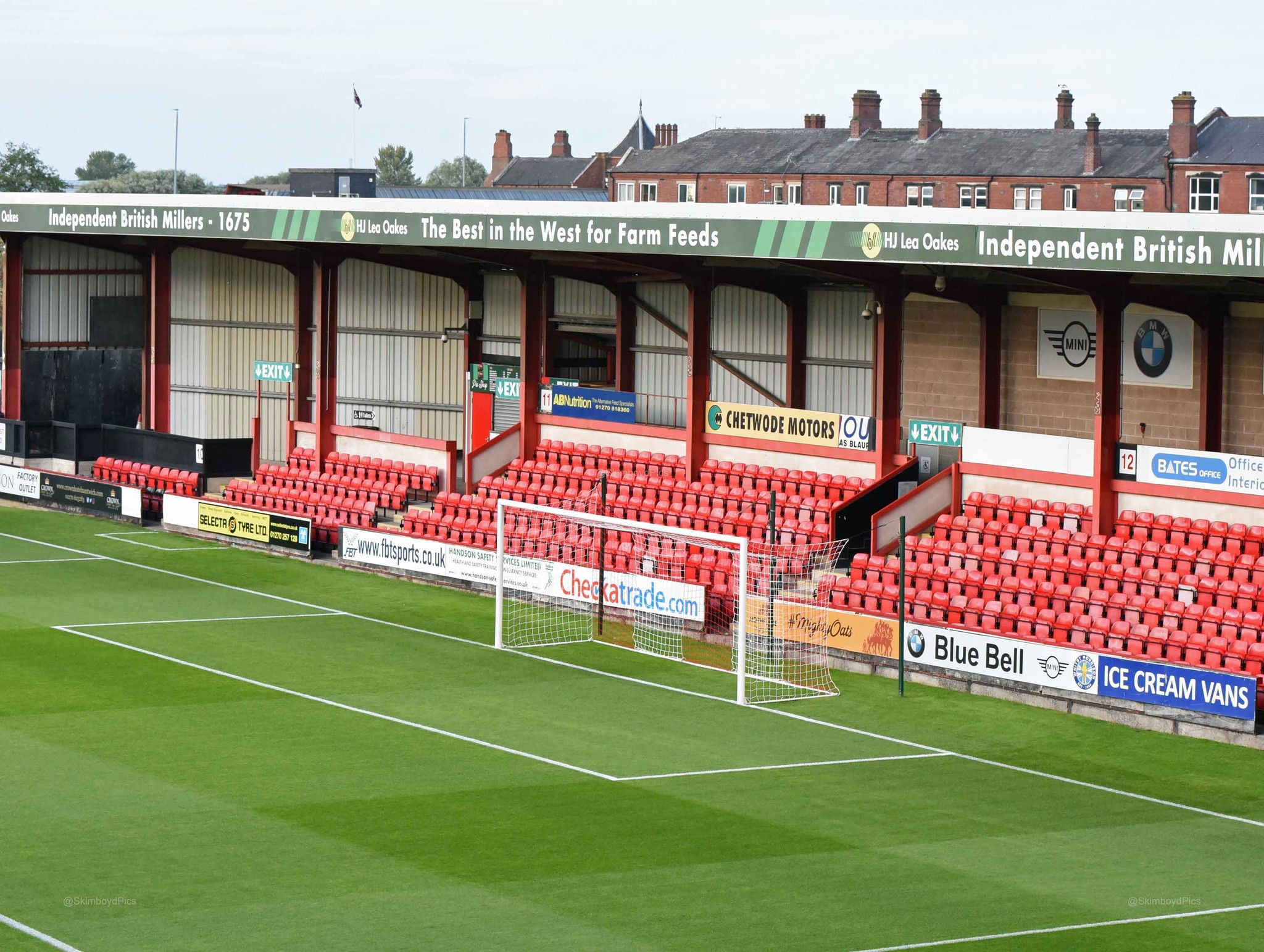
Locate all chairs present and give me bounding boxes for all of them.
[91,456,200,521]
[225,436,1264,713]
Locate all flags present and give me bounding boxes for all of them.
[354,88,362,108]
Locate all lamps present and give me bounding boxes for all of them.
[934,275,946,293]
[860,300,883,321]
[441,318,468,343]
